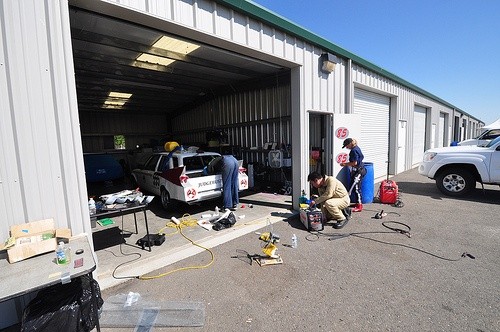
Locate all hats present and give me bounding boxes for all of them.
[341,138,353,148]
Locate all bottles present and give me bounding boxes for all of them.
[171,215,180,225]
[55,241,71,265]
[75,249,84,268]
[201,214,211,218]
[215,206,219,216]
[180,144,185,155]
[292,234,297,248]
[88,196,96,216]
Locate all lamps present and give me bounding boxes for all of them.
[320,52,337,75]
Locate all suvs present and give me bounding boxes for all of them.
[417,135,500,199]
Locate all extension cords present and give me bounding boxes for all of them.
[171,217,180,225]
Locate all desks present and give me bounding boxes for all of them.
[90,198,153,252]
[0,232,102,332]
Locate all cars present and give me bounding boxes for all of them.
[129,144,249,212]
[449,126,500,148]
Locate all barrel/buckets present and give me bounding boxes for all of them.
[348,162,374,203]
[380,180,398,204]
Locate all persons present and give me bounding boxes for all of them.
[202,155,239,213]
[307,170,352,229]
[340,138,367,212]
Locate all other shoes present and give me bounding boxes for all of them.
[352,204,362,212]
[334,219,348,229]
[229,205,238,210]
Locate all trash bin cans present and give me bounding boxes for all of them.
[19,274,104,332]
[346,161,375,204]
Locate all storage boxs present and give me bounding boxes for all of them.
[0,218,72,264]
